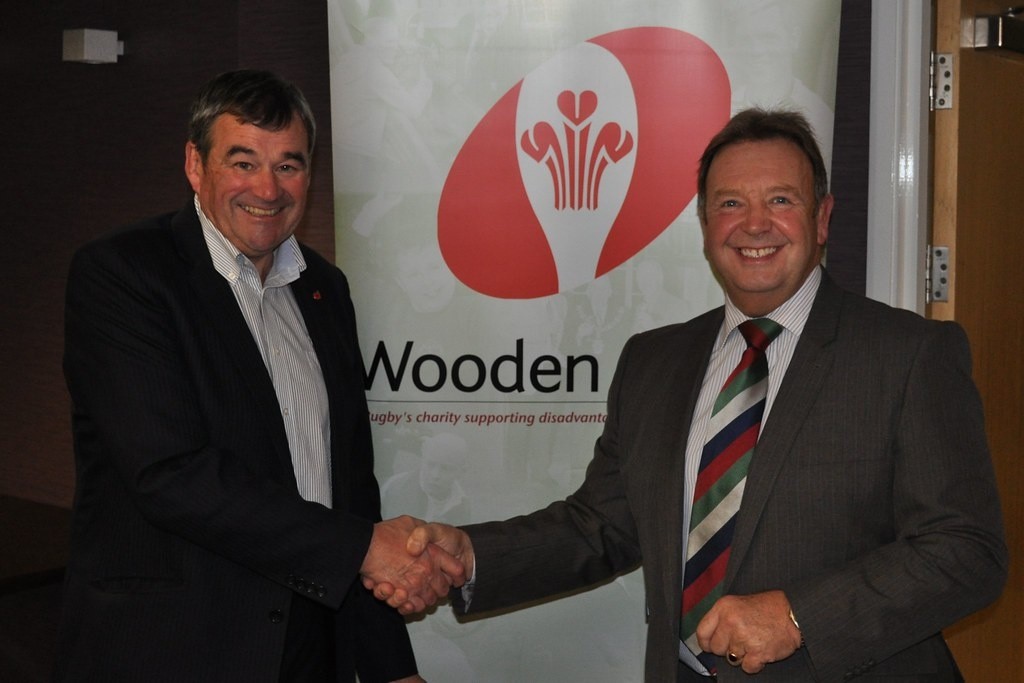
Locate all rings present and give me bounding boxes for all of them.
[729,649,743,662]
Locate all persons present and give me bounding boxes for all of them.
[363,108,1010,683]
[62,69,467,682]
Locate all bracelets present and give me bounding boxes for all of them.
[789,607,805,656]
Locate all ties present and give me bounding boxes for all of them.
[679,318,785,675]
